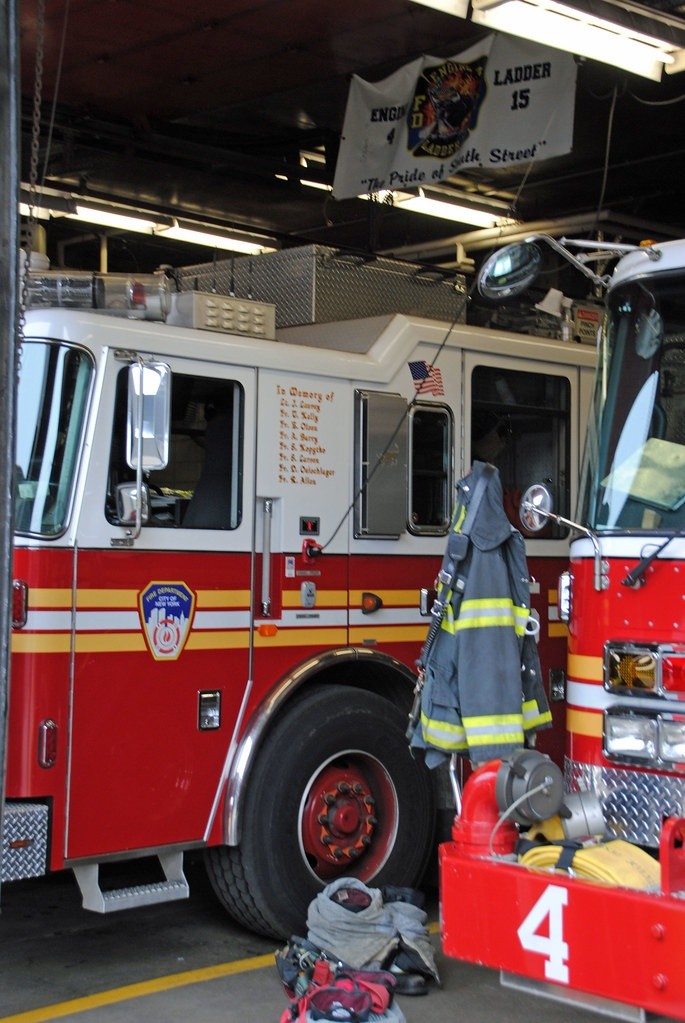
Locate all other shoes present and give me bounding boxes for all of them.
[390,970,429,995]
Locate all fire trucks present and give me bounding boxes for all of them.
[0,238,604,945]
[427,231,685,1023]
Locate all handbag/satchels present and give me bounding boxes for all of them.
[275,935,396,1014]
[281,972,371,1023]
